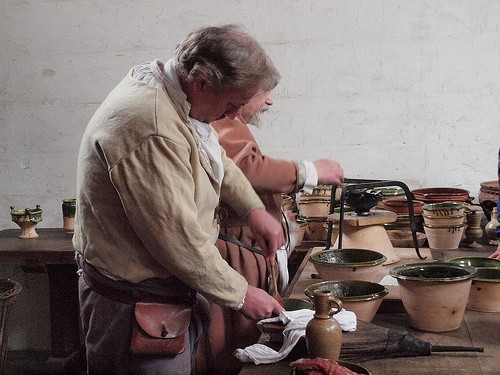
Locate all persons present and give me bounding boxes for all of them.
[195,56,344,375]
[72,23,286,375]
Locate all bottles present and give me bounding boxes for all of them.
[485,207,500,246]
[305,289,342,360]
[479,181,500,222]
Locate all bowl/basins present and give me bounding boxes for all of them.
[444,256,500,313]
[388,262,478,331]
[262,297,314,327]
[308,248,388,283]
[280,181,471,250]
[303,280,390,323]
[290,360,374,375]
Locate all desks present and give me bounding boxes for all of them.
[236,245,500,375]
[0,228,79,375]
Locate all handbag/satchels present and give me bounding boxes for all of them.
[129,302,190,357]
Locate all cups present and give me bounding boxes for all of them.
[61,199,76,234]
